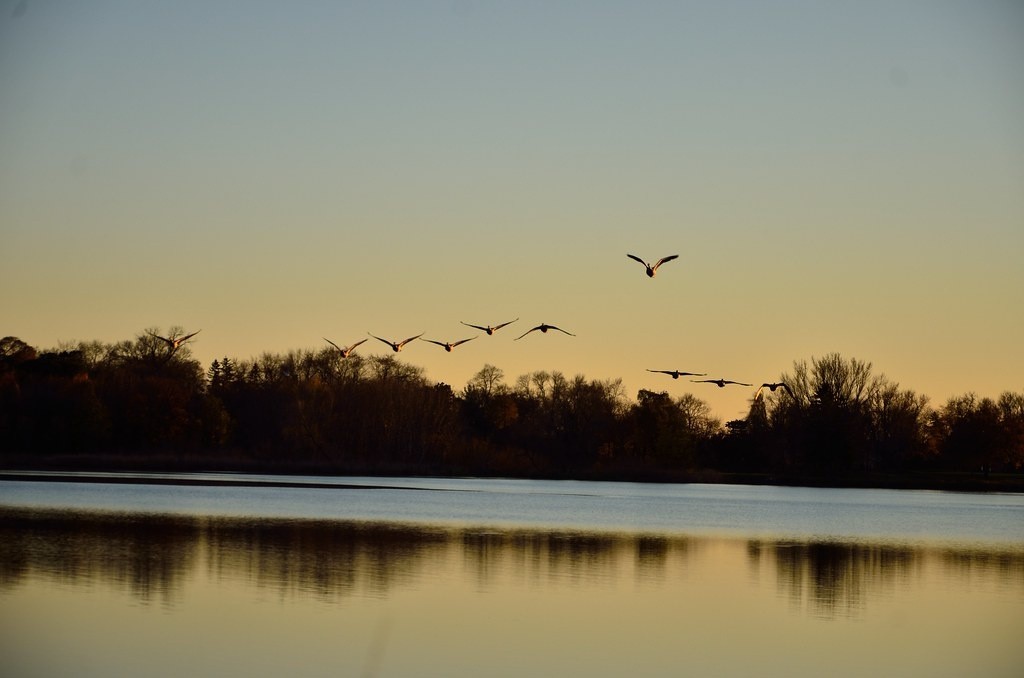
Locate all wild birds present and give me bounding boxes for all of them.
[626,253,680,278]
[148,328,202,350]
[321,316,578,359]
[644,368,798,403]
